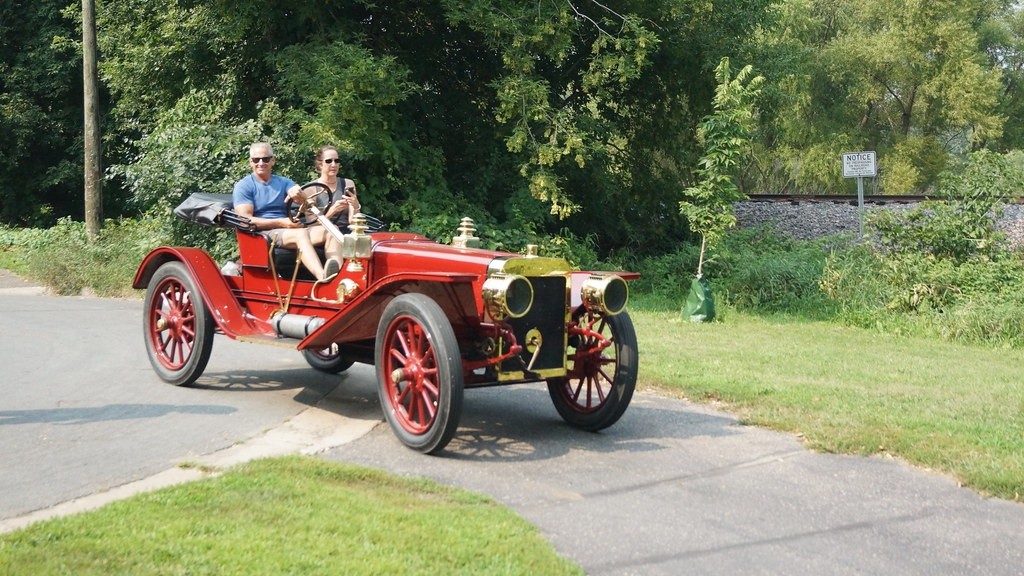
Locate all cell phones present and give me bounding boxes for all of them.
[345,186,354,197]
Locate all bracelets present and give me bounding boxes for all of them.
[353,204,361,210]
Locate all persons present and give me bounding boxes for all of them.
[233,142,344,284]
[299,146,362,234]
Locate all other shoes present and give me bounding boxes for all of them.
[322,258,340,283]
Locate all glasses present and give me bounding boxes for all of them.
[250,155,273,164]
[318,159,341,163]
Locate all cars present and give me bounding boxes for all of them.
[132,181,638,455]
